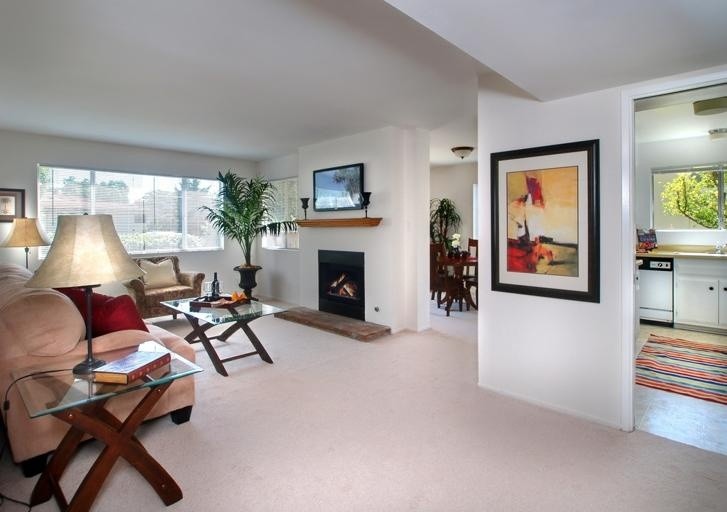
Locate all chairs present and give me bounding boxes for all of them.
[465,277,477,311]
[448,238,478,312]
[430,242,448,308]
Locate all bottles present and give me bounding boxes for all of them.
[212,272,219,295]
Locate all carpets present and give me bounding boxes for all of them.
[635,333,726,405]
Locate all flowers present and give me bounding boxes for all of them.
[450,233,461,248]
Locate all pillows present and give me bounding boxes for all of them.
[135,256,181,290]
[51,287,150,341]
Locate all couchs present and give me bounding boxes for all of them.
[0,261,197,478]
[121,255,205,320]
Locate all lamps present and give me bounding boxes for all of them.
[450,146,474,159]
[0,217,51,270]
[23,211,147,377]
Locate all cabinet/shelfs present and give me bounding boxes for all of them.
[638,270,673,328]
[673,255,726,335]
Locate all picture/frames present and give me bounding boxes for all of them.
[0,188,25,222]
[489,137,600,305]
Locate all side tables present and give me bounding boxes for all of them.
[8,340,205,512]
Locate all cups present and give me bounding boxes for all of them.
[203,282,212,301]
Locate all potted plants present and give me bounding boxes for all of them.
[193,167,296,305]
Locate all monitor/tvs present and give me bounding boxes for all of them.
[312,163,364,212]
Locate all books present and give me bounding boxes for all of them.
[91,352,171,385]
